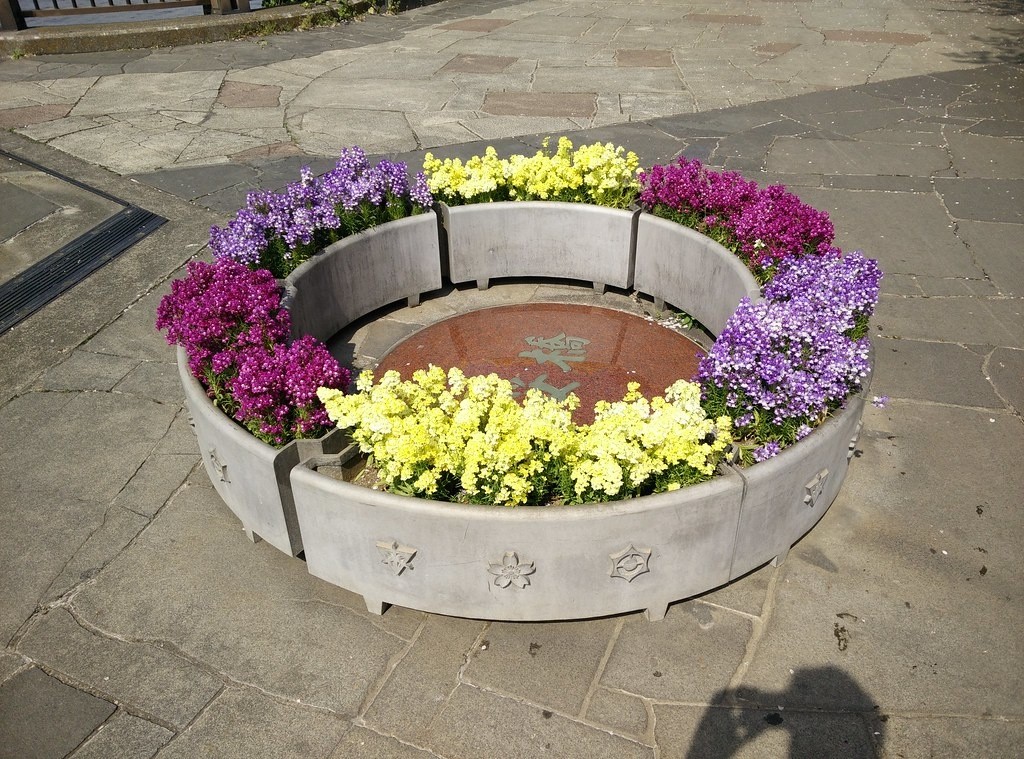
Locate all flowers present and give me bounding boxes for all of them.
[154,250,353,446]
[207,143,435,276]
[317,353,736,506]
[422,134,647,209]
[638,155,844,287]
[687,244,885,463]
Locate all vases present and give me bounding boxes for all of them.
[273,207,444,346]
[721,318,876,584]
[174,335,354,560]
[290,439,746,624]
[433,192,641,294]
[633,205,772,343]
